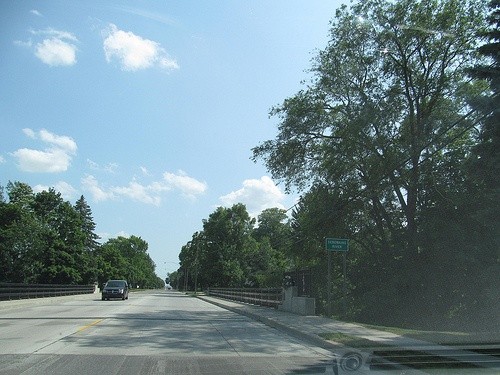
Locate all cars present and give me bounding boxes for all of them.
[101,279,129,300]
